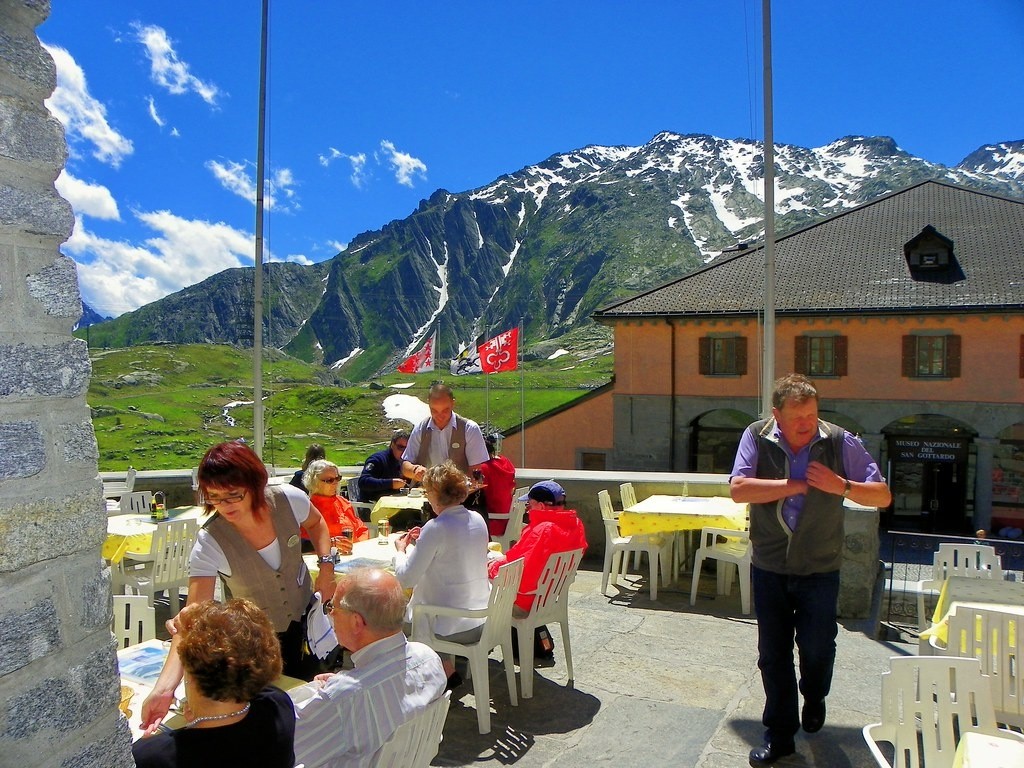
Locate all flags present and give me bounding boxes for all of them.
[450,329,486,376]
[396,329,436,373]
[479,328,520,373]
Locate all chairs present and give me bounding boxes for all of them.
[346,476,392,539]
[371,485,583,768]
[99,463,293,651]
[689,510,755,615]
[864,542,1024,768]
[597,482,687,602]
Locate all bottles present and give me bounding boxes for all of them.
[151,499,168,521]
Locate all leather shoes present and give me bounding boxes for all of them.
[802,696,826,733]
[749,734,795,766]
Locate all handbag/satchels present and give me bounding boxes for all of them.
[511,625,554,659]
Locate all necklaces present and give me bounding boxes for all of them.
[185,702,252,729]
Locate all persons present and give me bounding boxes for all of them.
[293,567,447,768]
[394,459,493,691]
[489,479,588,619]
[239,434,245,443]
[300,458,369,554]
[139,438,339,737]
[129,597,295,767]
[288,445,326,495]
[973,529,992,565]
[480,433,516,536]
[356,431,409,523]
[400,385,490,482]
[728,372,892,768]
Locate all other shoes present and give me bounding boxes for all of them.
[447,671,462,689]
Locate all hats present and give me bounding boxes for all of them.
[517,480,566,506]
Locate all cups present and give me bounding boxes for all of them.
[377,519,389,545]
[162,639,172,666]
[341,526,353,555]
[399,487,420,496]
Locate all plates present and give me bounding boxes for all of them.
[408,493,422,498]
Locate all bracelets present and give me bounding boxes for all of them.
[315,554,336,564]
[840,478,852,499]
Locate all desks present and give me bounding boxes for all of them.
[267,475,360,487]
[299,533,502,672]
[951,732,1024,768]
[117,640,309,747]
[618,494,746,597]
[919,601,1024,677]
[101,506,218,619]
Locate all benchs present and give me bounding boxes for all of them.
[882,579,942,621]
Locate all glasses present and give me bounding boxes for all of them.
[202,487,248,505]
[393,439,406,451]
[317,474,342,484]
[323,598,366,625]
